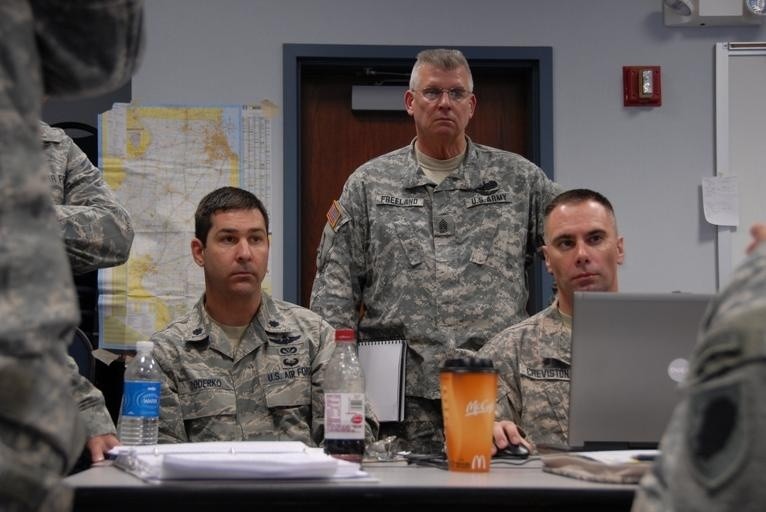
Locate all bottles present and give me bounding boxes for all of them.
[120,337,160,443]
[323,326,367,464]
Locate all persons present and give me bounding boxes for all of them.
[0,1,146,512]
[118,185,381,460]
[630,222,766,511]
[308,50,564,454]
[476,189,626,454]
[37,120,135,272]
[61,351,125,463]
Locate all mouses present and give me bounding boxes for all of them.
[493,441,531,460]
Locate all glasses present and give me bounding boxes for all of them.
[411,85,473,104]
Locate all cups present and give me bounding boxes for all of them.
[438,355,496,472]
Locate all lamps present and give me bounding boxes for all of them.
[662,0,766,30]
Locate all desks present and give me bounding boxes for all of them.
[61,448,645,511]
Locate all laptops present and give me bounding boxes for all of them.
[537,290,716,454]
